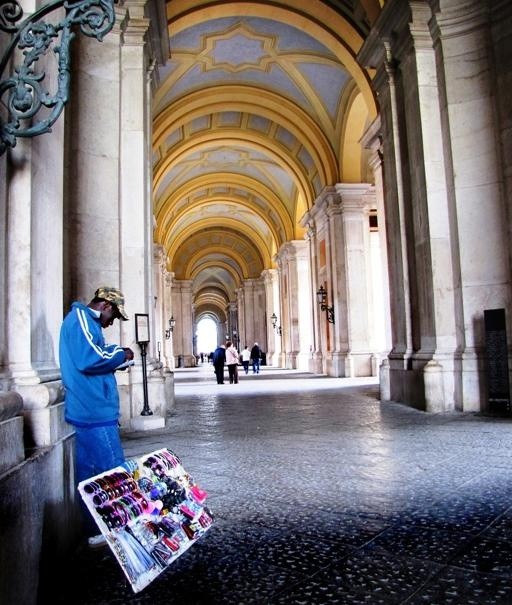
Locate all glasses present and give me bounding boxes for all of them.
[142,446,181,476]
[84,460,152,531]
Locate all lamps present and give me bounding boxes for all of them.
[316,283,336,325]
[270,312,282,336]
[232,326,239,343]
[166,315,176,340]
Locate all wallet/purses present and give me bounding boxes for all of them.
[183,520,199,540]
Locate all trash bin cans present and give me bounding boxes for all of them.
[178,355,184,369]
[262,353,266,365]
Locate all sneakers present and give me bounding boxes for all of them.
[88,533,107,548]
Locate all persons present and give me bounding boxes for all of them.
[199,341,266,385]
[57,286,136,572]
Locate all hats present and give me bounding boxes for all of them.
[95,286,128,321]
